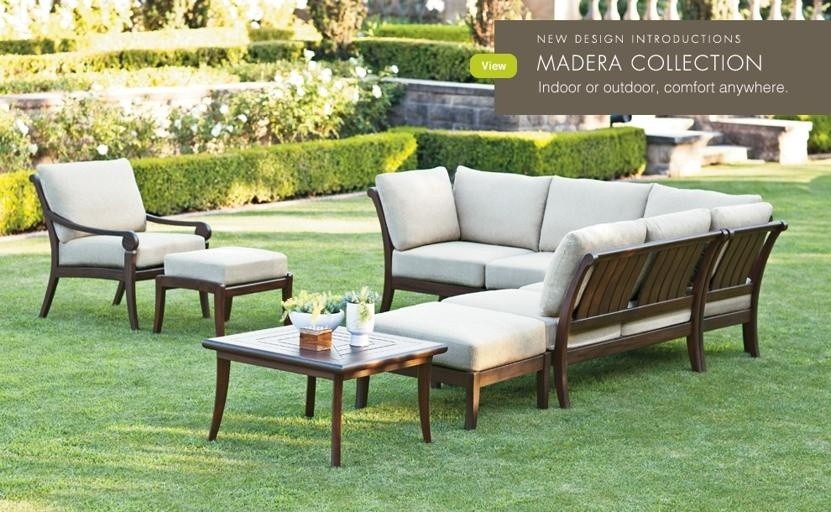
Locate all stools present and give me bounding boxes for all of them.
[152,248,294,338]
[354,299,549,431]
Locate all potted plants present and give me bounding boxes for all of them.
[277,284,381,348]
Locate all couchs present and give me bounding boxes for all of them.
[367,163,789,412]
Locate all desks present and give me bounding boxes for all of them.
[200,325,449,467]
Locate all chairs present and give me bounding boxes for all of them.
[28,155,214,331]
